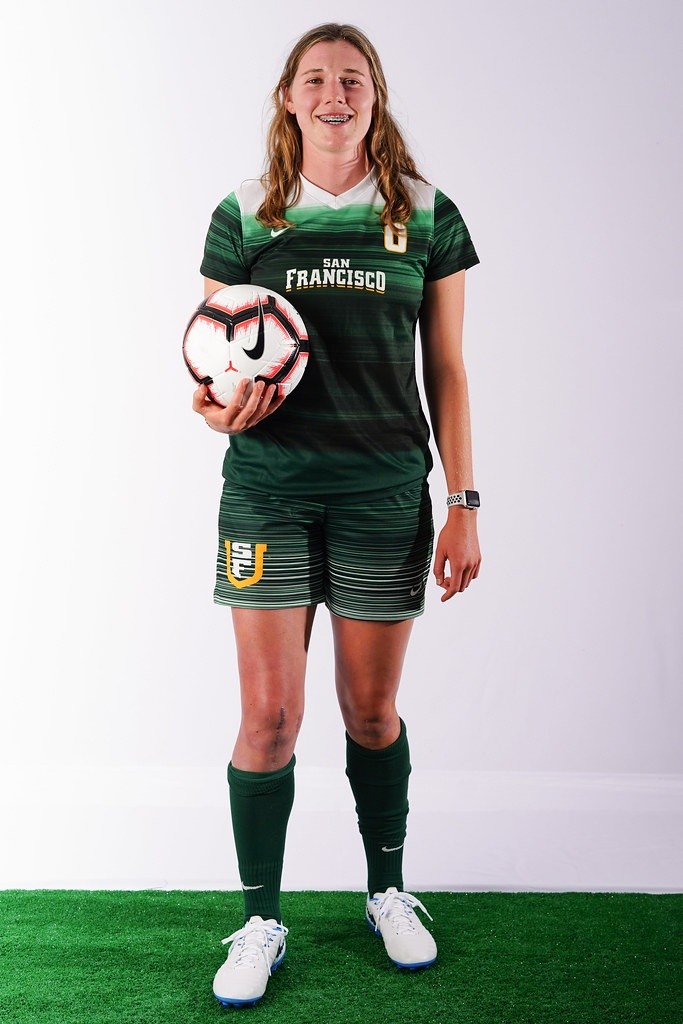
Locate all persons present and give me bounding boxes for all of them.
[190,20,483,1009]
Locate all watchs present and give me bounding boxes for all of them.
[446,490,480,509]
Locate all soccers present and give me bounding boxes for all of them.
[181,283,311,409]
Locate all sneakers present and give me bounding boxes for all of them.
[362,886,442,968]
[210,922,292,1005]
